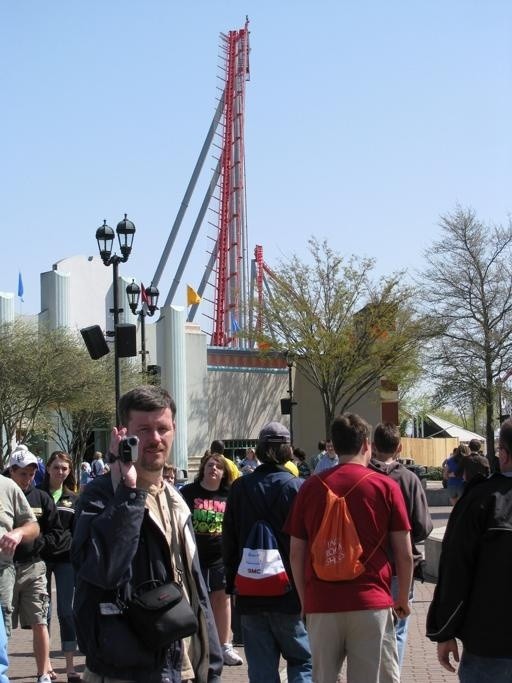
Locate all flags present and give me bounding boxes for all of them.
[232,311,240,333]
[18,270,24,297]
[187,284,200,305]
[141,281,151,306]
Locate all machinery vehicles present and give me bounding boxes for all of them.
[396,456,430,484]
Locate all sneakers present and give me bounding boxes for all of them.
[37,673,51,682]
[221,643,243,665]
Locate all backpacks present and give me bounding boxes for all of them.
[233,475,295,596]
[311,471,387,581]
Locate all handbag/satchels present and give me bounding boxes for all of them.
[123,579,198,650]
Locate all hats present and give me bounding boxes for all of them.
[259,422,291,443]
[9,445,39,472]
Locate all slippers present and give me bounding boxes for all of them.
[47,669,56,679]
[67,672,79,680]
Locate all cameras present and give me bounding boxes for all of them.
[119,435,140,463]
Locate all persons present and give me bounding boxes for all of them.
[73,384,223,682]
[163,411,510,681]
[1,445,110,681]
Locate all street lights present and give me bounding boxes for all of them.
[496,374,506,425]
[80,214,138,430]
[280,349,298,448]
[124,278,160,387]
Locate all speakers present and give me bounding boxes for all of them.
[115,324,136,357]
[281,399,291,414]
[80,325,110,360]
